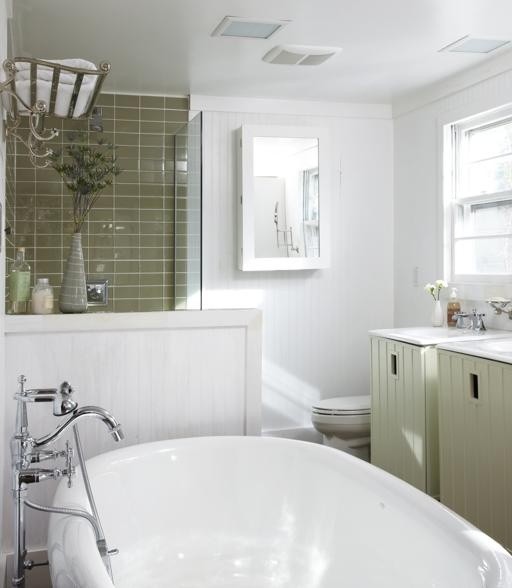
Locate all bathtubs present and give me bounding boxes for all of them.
[47,436,511,588]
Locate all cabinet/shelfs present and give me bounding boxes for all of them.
[368,335,512,553]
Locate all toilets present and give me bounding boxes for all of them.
[312,395,371,463]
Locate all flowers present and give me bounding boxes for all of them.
[424,279,448,301]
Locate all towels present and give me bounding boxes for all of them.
[11,56,99,120]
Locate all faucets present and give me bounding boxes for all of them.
[18,405,124,451]
[486,297,511,320]
[451,308,486,330]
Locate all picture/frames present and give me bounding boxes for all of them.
[86,279,109,306]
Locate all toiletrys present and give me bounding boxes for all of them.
[447,287,461,327]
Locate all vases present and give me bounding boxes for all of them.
[431,300,444,327]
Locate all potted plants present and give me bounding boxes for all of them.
[49,128,123,313]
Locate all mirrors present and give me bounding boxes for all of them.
[235,123,332,273]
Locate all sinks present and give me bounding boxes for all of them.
[480,338,512,356]
[391,326,465,338]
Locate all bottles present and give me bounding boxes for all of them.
[32,277,54,316]
[9,246,31,314]
